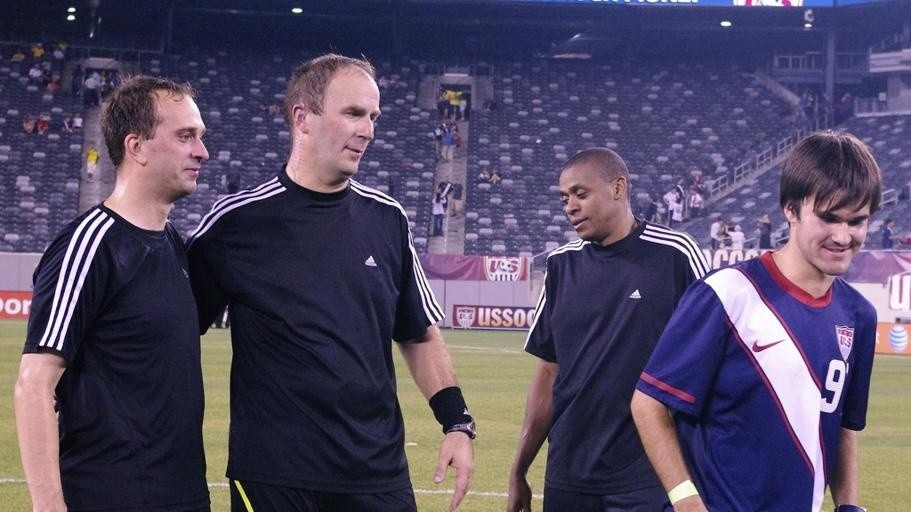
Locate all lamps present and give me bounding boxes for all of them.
[559,16,585,40]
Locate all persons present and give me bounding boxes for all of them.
[645,174,896,250]
[86,140,100,182]
[185,53,477,512]
[11,38,121,136]
[225,102,279,195]
[631,130,883,512]
[14,75,210,512]
[507,147,710,512]
[429,88,504,238]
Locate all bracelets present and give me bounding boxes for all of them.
[668,480,700,506]
[428,386,472,433]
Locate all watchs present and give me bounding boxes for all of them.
[444,417,476,439]
